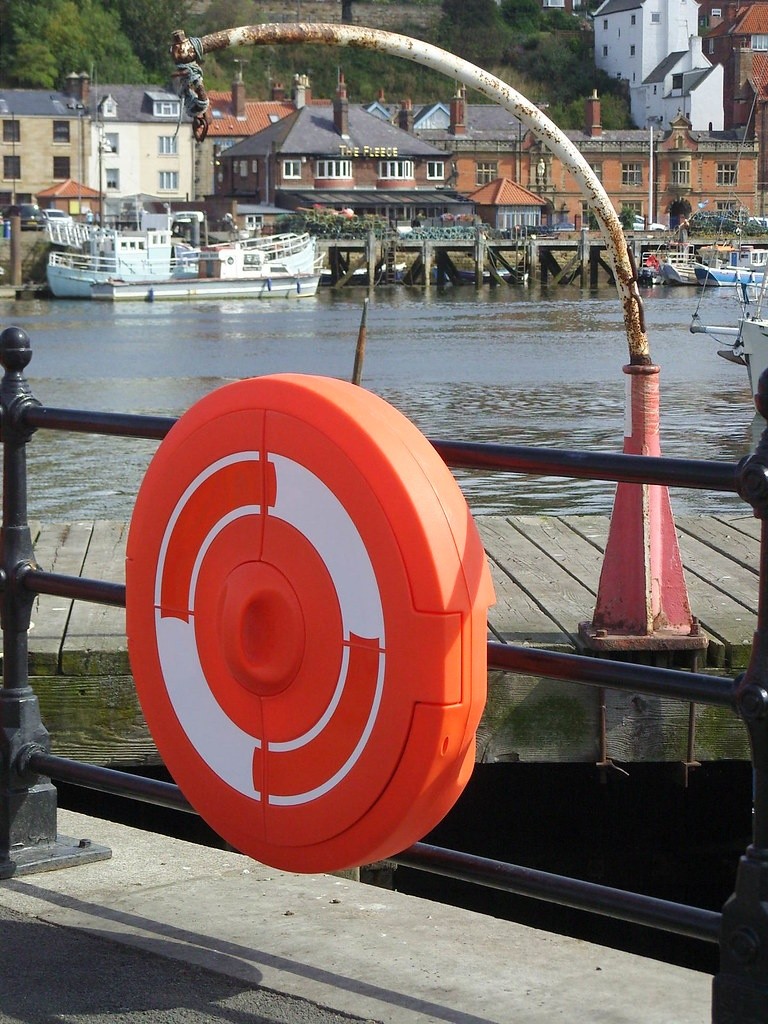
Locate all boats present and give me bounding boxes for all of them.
[688,56,768,422]
[636,228,768,292]
[318,259,516,286]
[43,208,330,302]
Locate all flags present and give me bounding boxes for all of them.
[100,128,112,153]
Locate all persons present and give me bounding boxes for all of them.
[659,254,671,270]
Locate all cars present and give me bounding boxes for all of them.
[41,209,74,227]
[503,225,550,238]
[616,215,668,232]
[3,205,47,231]
[548,222,589,232]
